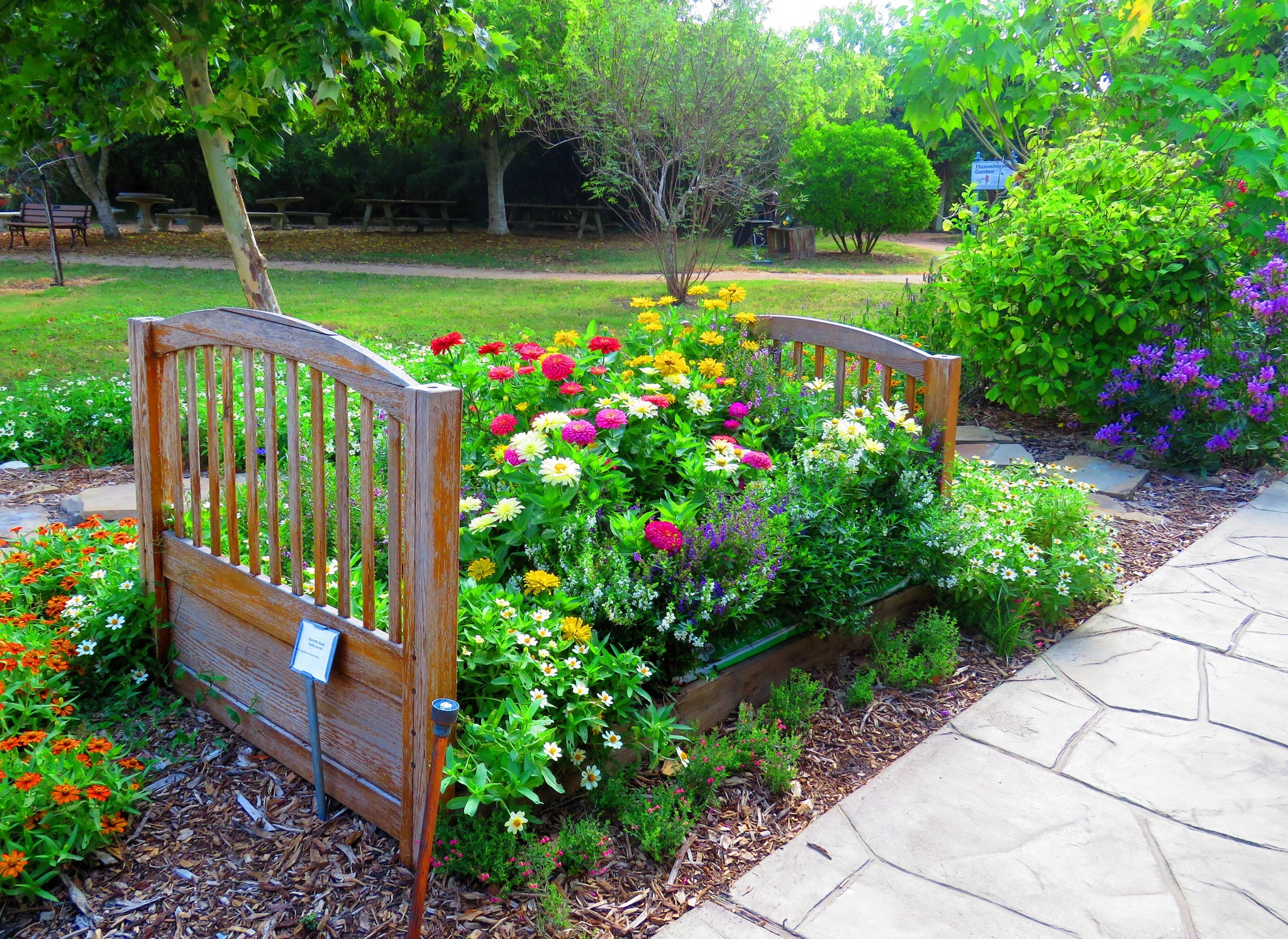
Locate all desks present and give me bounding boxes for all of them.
[255,196,304,229]
[355,198,457,238]
[118,192,165,223]
[504,201,615,240]
[115,196,174,233]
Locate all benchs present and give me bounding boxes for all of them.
[284,210,330,229]
[339,216,373,221]
[483,218,591,226]
[4,201,93,250]
[246,211,284,230]
[154,212,209,233]
[590,222,623,229]
[167,208,196,225]
[373,216,468,222]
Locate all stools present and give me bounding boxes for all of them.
[111,206,126,225]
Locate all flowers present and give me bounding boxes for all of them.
[229,274,962,832]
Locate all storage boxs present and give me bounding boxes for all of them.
[766,222,816,260]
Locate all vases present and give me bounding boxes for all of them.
[552,554,938,803]
